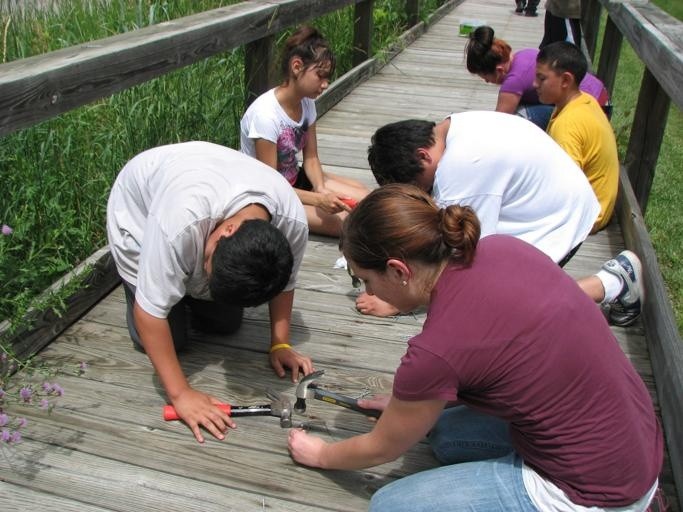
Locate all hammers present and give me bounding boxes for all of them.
[163,369,383,428]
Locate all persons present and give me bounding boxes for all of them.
[531,38,622,237]
[283,179,664,512]
[463,22,614,136]
[238,21,376,240]
[537,0,586,52]
[105,139,317,444]
[351,111,645,326]
[513,0,542,17]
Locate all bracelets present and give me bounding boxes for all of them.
[269,342,292,354]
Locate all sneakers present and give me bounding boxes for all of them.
[515,6,539,17]
[600,249,645,328]
